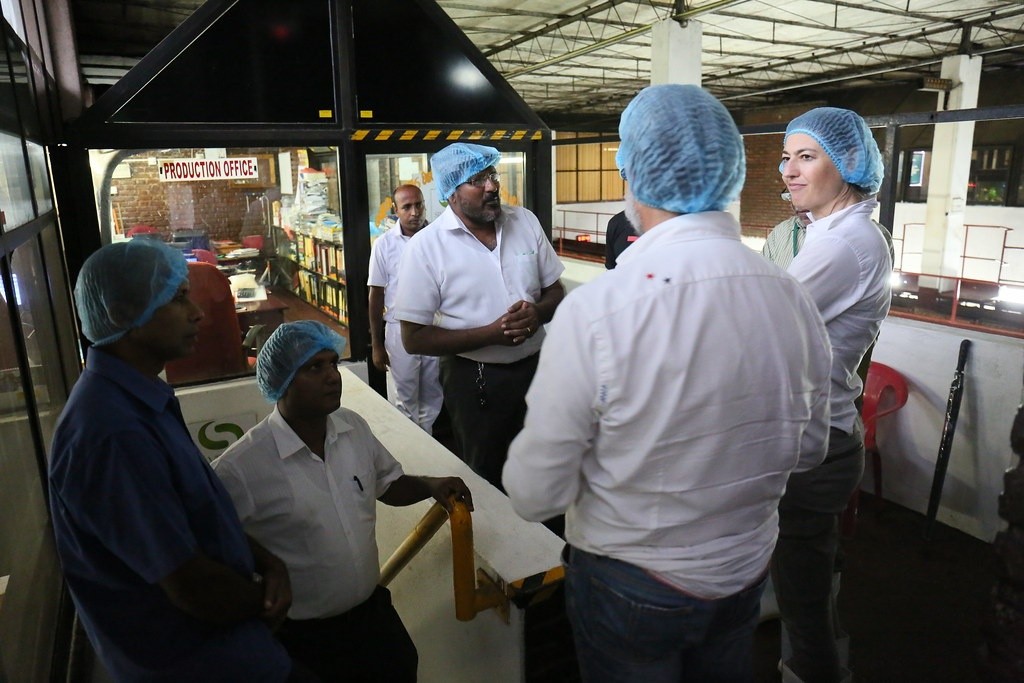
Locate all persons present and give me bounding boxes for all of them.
[604,142,639,270]
[765,208,895,345]
[209,320,474,683]
[503,84,832,682]
[393,143,566,541]
[770,108,892,683]
[367,185,443,437]
[48,242,321,683]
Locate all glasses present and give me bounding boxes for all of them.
[468,174,501,187]
[780,188,791,201]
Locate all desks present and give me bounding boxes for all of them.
[218,255,261,266]
[234,293,289,348]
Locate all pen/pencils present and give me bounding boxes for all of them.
[353,475,363,492]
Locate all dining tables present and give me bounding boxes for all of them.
[126,225,264,268]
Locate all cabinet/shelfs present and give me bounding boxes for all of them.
[272,225,349,328]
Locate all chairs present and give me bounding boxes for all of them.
[835,362,908,545]
[164,261,267,384]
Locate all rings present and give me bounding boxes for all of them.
[527,327,531,332]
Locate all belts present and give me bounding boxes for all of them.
[449,357,488,368]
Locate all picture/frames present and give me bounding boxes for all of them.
[227,154,276,188]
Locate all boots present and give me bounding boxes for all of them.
[833,630,853,666]
[777,657,852,683]
